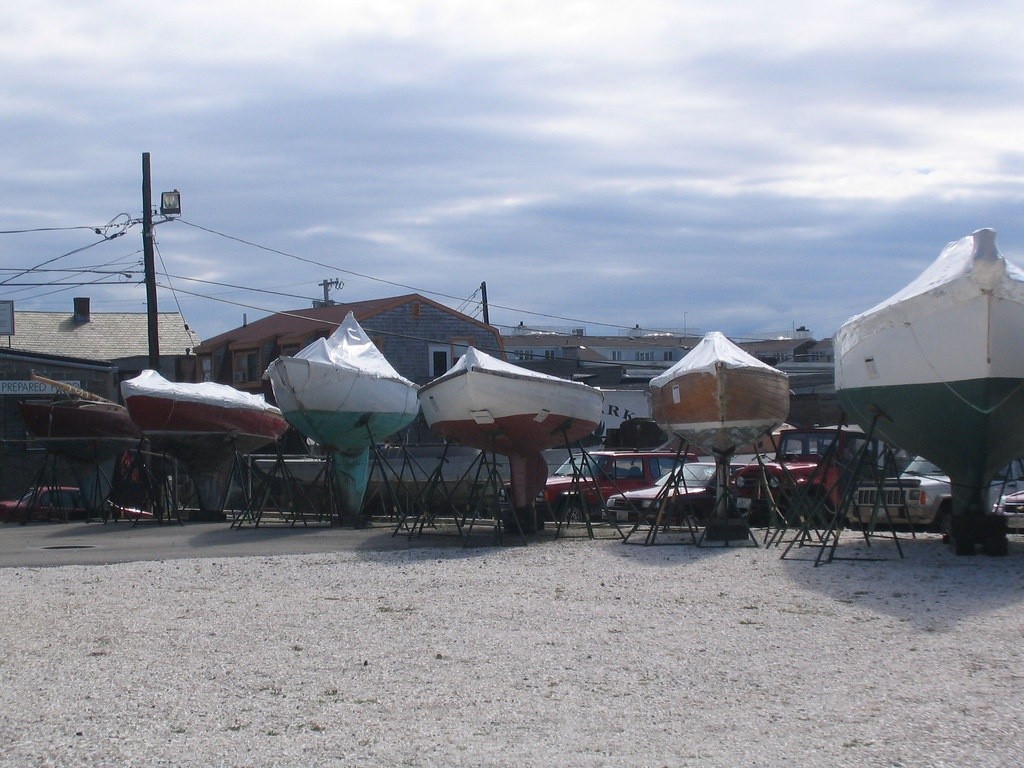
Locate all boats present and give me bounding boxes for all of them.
[646,331,792,524]
[120,371,289,518]
[417,346,600,507]
[268,305,421,529]
[19,367,147,466]
[832,224,1024,547]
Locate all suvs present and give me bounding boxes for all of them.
[855,446,1024,534]
[733,425,884,534]
[497,446,700,525]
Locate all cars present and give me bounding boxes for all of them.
[0,487,156,521]
[603,459,749,525]
